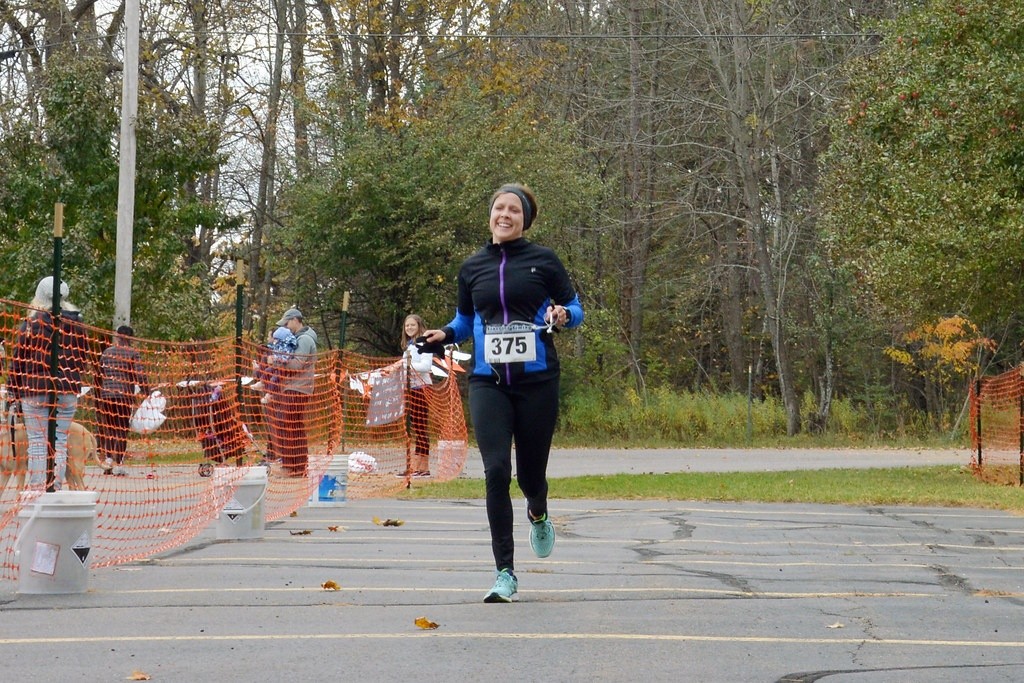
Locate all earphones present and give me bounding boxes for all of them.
[546,326,553,333]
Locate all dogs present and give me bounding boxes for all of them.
[0,421,112,499]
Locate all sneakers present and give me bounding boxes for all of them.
[525,498,554,557]
[484,570,518,602]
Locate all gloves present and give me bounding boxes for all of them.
[415,334,445,358]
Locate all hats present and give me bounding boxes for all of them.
[272,327,290,339]
[275,309,301,326]
[35,276,70,307]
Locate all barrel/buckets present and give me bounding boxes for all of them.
[307,454,350,508]
[438,440,468,478]
[13,490,99,595]
[213,466,268,541]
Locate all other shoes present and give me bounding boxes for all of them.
[114,467,130,476]
[261,393,274,403]
[397,470,411,478]
[251,381,264,390]
[413,471,430,478]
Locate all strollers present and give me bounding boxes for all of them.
[177,374,275,477]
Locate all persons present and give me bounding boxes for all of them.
[98,326,152,475]
[8,276,88,492]
[250,309,318,479]
[423,183,584,603]
[380,314,434,478]
